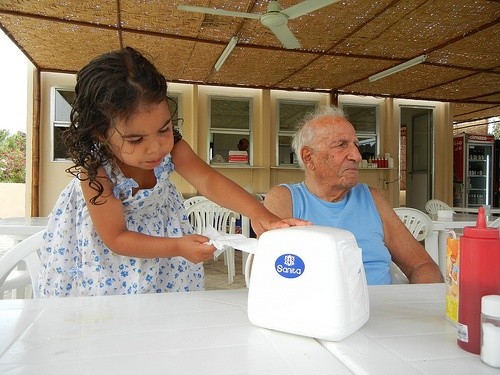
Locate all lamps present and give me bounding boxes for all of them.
[215,36,238,72]
[368,54,431,85]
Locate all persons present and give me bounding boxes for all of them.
[35,47,313,298]
[263,107,445,284]
[238,139,249,151]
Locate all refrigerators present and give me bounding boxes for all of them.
[454,132,495,215]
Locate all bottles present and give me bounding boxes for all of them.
[468,193,484,204]
[469,178,472,189]
[469,148,486,160]
[457,206,500,354]
[479,294,500,367]
[469,164,483,175]
[368,155,388,168]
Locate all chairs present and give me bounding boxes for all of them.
[182,194,240,287]
[389,205,435,246]
[425,199,459,215]
[0,227,49,298]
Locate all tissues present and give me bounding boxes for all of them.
[201,220,371,342]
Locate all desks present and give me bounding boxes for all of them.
[452,206,500,214]
[0,282,497,375]
[0,214,51,236]
[424,212,500,278]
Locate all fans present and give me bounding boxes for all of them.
[177,0,344,51]
